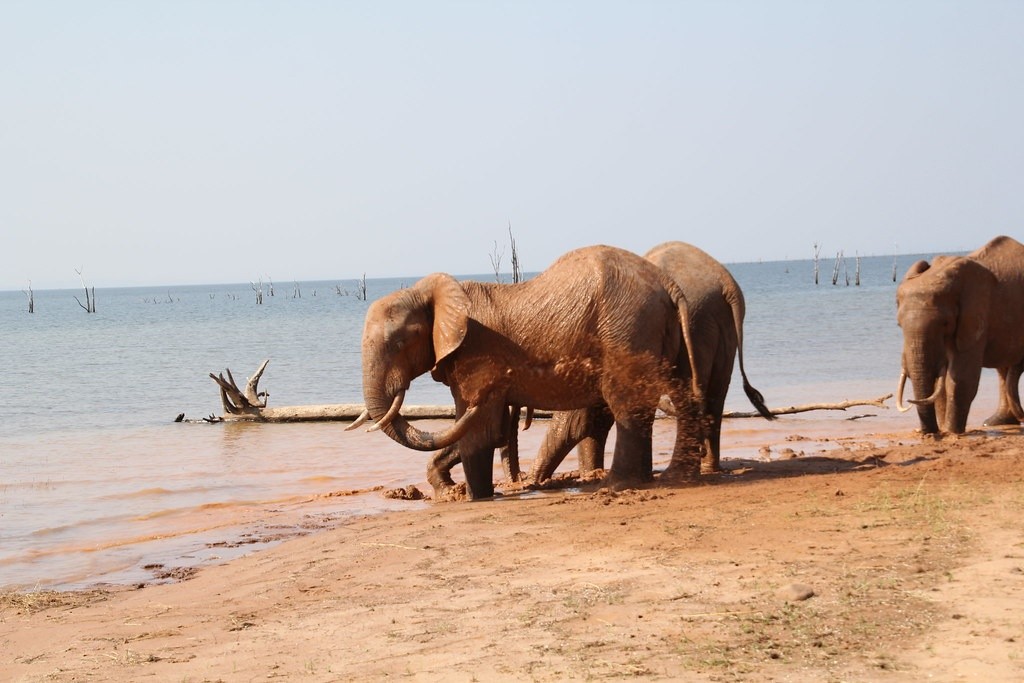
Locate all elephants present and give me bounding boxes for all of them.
[343,240,776,506]
[895,236,1024,435]
